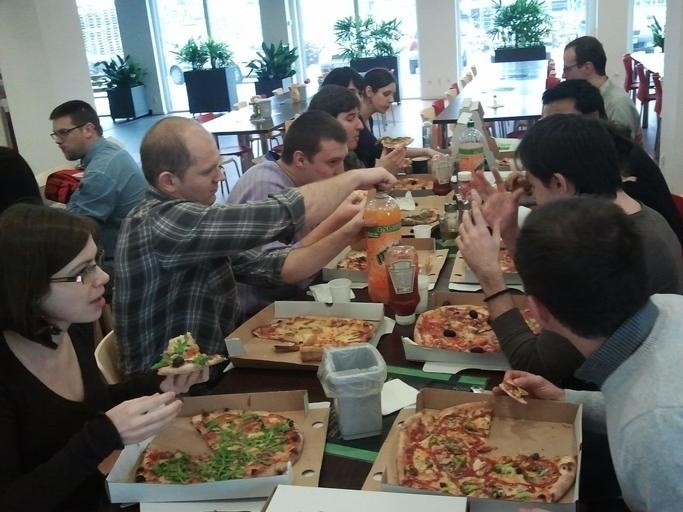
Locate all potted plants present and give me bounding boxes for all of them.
[330,10,404,108]
[250,40,297,94]
[169,32,240,115]
[482,0,553,63]
[90,53,151,123]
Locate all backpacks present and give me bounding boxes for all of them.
[44,168,87,203]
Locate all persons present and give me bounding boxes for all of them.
[482,195,683,512]
[111,116,398,395]
[49,101,149,303]
[0,146,44,214]
[455,113,683,391]
[562,36,643,145]
[0,199,210,512]
[504,79,683,245]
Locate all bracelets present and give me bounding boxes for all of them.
[483,287,510,303]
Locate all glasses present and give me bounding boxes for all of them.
[49,249,107,285]
[49,123,88,141]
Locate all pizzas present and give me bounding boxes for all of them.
[251,316,376,362]
[150,332,228,376]
[499,250,519,274]
[413,301,543,354]
[397,381,576,503]
[375,136,414,151]
[135,408,304,484]
[393,177,433,190]
[337,250,368,272]
[400,208,438,224]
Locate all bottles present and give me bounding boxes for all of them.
[363,184,403,304]
[289,83,300,103]
[383,243,421,325]
[457,121,487,202]
[443,202,458,235]
[492,95,498,106]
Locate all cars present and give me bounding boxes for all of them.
[551,28,577,49]
[168,42,263,85]
[575,8,656,58]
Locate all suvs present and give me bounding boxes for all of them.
[407,24,468,75]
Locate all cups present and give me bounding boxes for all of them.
[404,156,428,175]
[328,277,353,304]
[414,274,431,315]
[430,155,453,195]
[256,99,271,119]
[413,224,433,240]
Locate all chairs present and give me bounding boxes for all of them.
[420,53,560,149]
[195,86,322,196]
[624,52,666,161]
[91,329,119,384]
